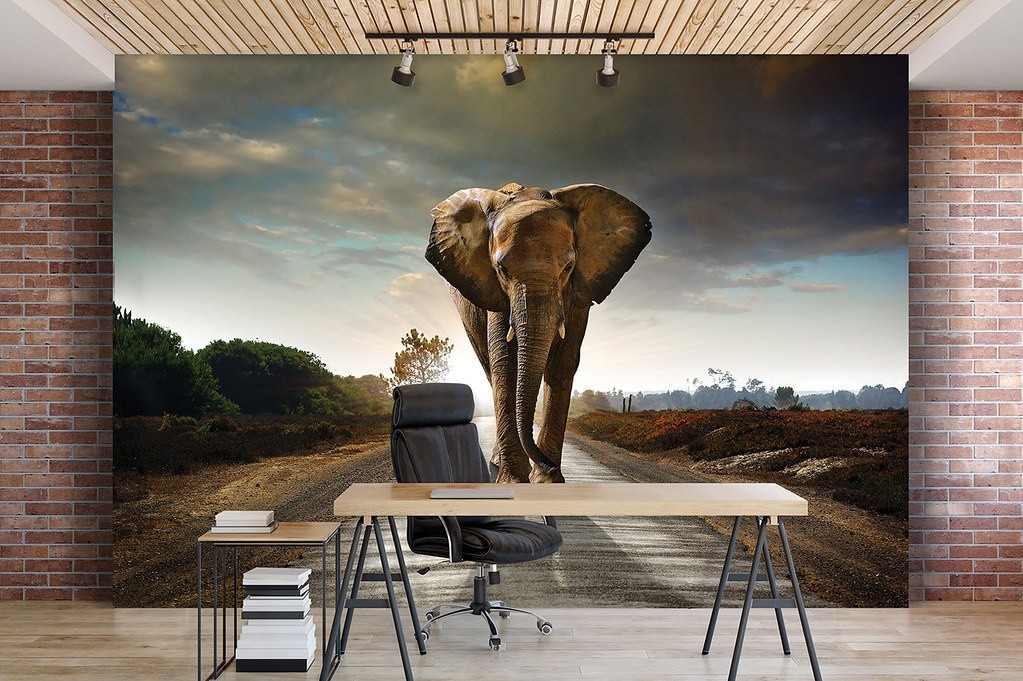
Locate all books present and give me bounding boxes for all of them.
[235,567,316,673]
[210,510,280,534]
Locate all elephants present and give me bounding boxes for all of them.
[425,182,652,482]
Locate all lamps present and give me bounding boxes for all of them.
[500,37,525,87]
[595,38,618,89]
[390,36,417,87]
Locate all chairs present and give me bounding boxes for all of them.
[391,383,565,649]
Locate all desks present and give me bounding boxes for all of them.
[318,481,822,681]
[196,522,341,679]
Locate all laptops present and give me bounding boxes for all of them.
[430,489,515,499]
